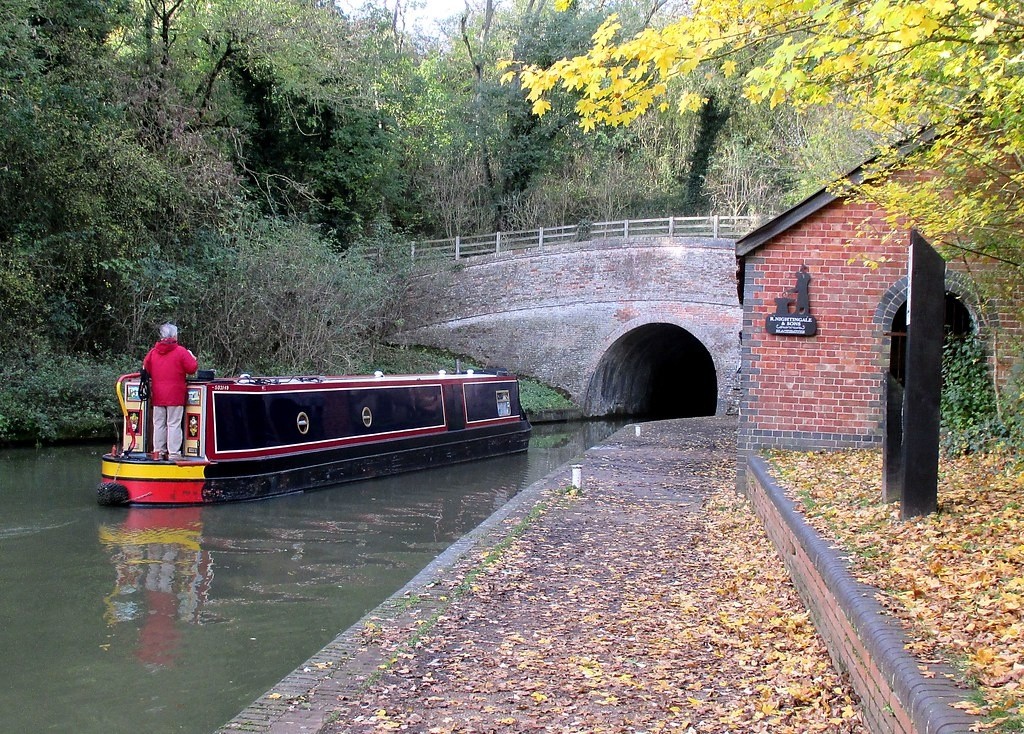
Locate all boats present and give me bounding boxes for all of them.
[97,357,534,508]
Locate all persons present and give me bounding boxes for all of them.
[142,323,199,462]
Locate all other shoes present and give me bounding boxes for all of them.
[159,453,164,460]
[169,453,183,461]
